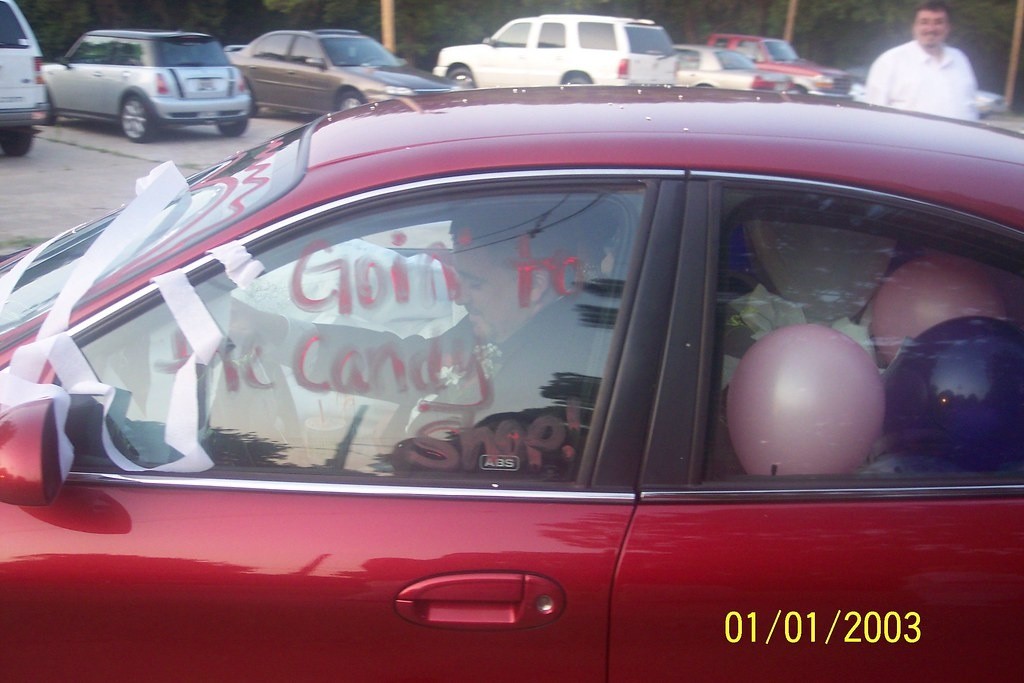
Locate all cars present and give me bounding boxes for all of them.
[227,29,467,118]
[39,29,253,144]
[848,68,1008,118]
[673,45,794,96]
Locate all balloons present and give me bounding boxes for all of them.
[727,221,1024,477]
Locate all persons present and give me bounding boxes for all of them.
[231,199,623,482]
[865,0,978,123]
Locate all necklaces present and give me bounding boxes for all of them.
[472,289,571,380]
[577,263,604,284]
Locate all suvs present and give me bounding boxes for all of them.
[0,0,53,154]
[705,32,855,99]
[432,15,679,96]
[3,87,1024,683]
